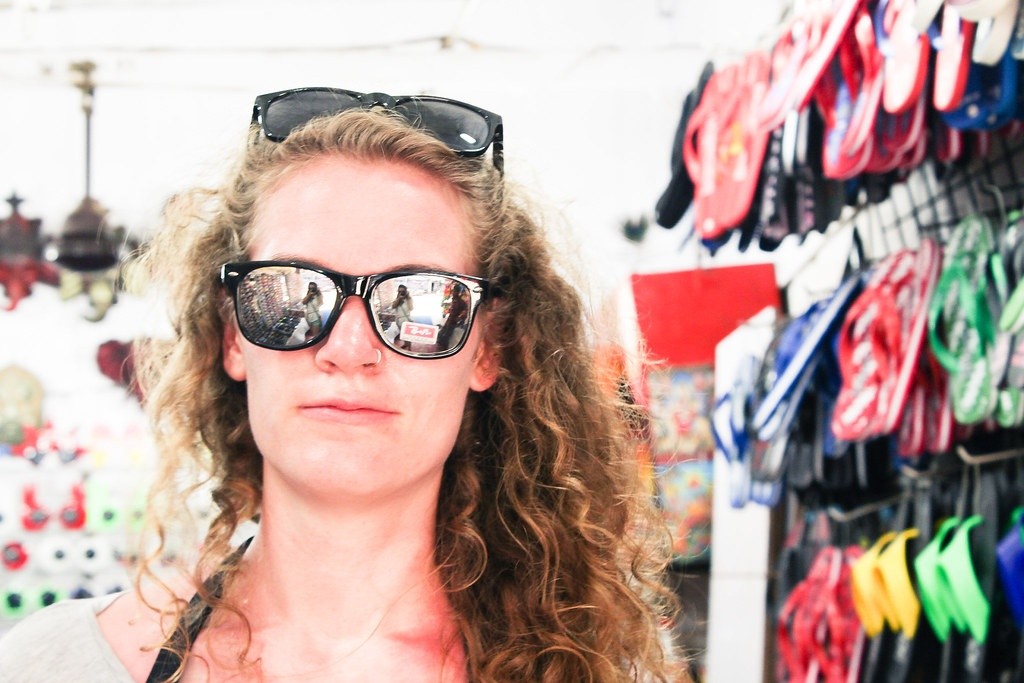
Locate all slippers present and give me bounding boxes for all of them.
[624,0,1024,683]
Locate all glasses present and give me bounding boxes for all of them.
[219,256,489,360]
[251,87,506,180]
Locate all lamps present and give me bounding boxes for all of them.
[47,56,118,273]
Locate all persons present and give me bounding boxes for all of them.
[2,88,692,683]
[302,282,324,337]
[391,285,413,352]
[435,285,467,351]
